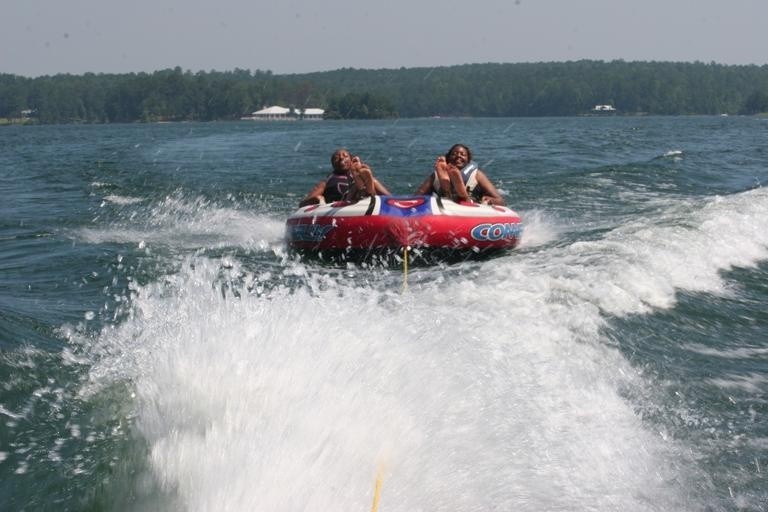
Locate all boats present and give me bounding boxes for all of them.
[285,192,523,269]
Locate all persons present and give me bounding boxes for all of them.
[299,148,393,207]
[416,143,506,206]
